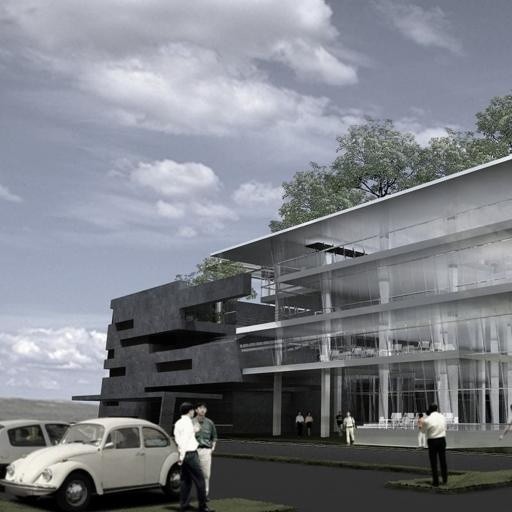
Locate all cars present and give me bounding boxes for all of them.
[0,417,182,512]
